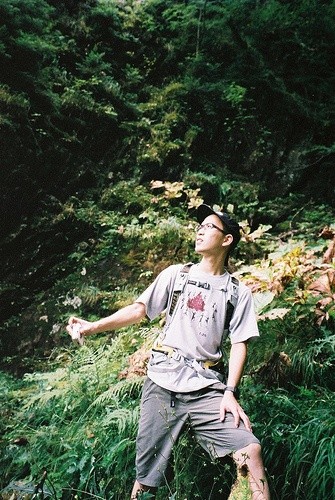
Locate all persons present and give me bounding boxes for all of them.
[67,203,273,500]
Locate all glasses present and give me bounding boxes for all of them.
[196,224,228,235]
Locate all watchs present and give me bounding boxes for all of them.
[223,386,239,394]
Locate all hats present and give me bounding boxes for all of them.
[195,203,242,242]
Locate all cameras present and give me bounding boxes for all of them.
[72,322,85,351]
